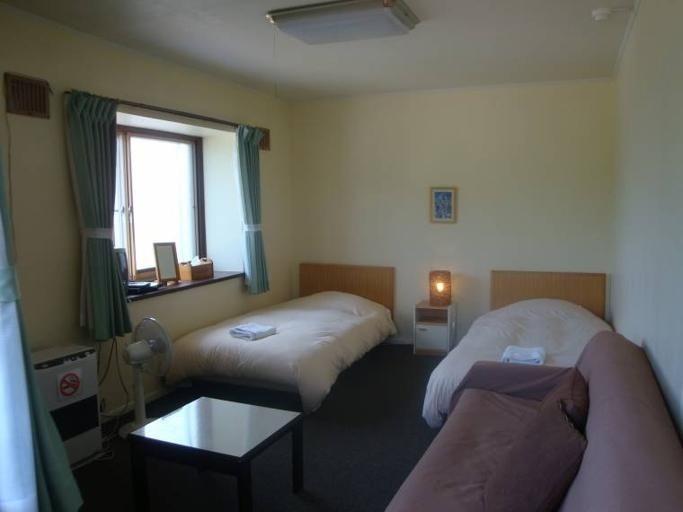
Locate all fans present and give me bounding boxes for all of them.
[118,316,174,440]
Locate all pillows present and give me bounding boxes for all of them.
[540,365,589,431]
[481,393,588,512]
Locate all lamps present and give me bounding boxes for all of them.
[265,0,420,46]
[429,270,451,307]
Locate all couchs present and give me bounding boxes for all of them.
[384,330,683,512]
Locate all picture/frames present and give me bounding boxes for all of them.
[430,187,457,224]
[153,241,181,287]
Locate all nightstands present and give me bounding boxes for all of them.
[413,301,457,357]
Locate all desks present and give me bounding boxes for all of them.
[126,396,305,511]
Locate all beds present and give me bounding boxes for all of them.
[421,269,614,429]
[164,261,398,416]
